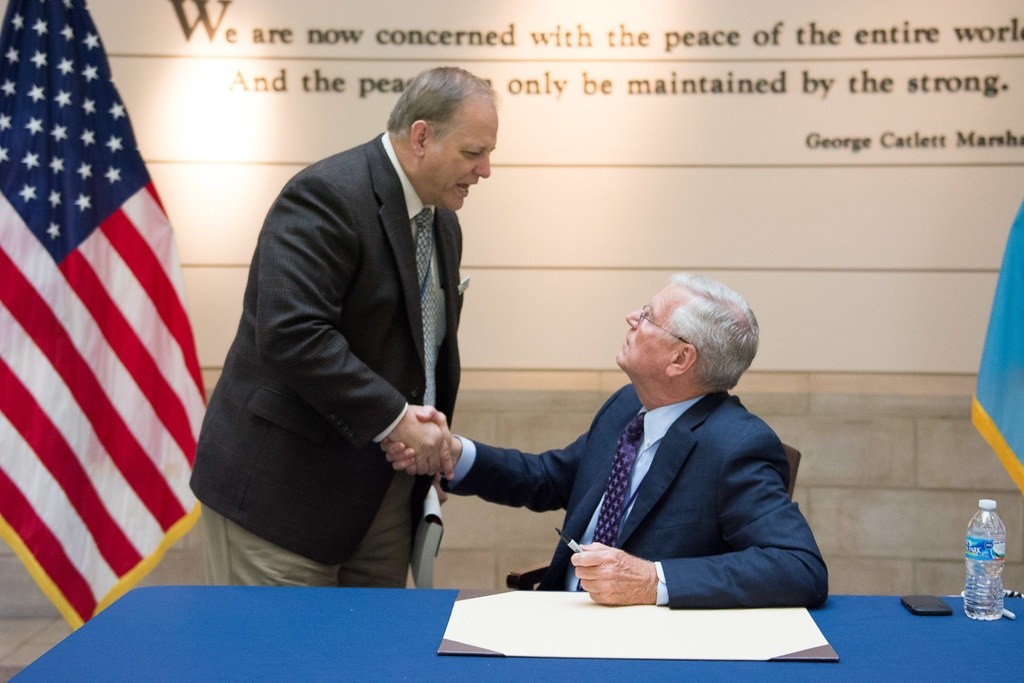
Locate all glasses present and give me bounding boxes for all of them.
[638,306,698,361]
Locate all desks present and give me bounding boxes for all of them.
[10,586,1024,683]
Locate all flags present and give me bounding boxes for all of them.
[0,1,211,633]
[971,200,1024,495]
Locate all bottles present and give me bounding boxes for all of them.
[964,499,1006,620]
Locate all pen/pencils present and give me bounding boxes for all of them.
[961,591,1016,620]
[555,528,583,552]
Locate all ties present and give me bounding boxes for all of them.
[414,207,437,409]
[576,412,646,592]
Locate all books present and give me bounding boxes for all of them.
[410,485,444,588]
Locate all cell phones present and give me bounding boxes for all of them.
[900,595,953,616]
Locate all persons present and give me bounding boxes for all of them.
[189,67,498,588]
[381,274,829,610]
